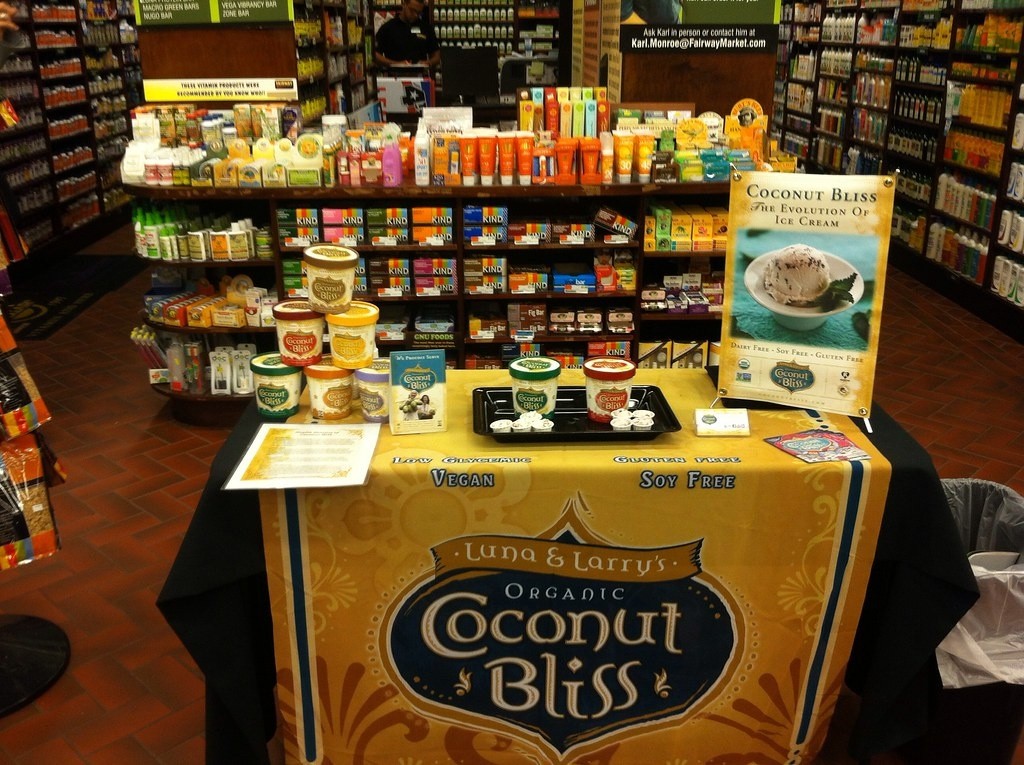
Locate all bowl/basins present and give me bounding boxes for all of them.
[741,245,866,332]
[490,410,554,433]
[610,407,655,430]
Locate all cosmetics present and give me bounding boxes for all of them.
[127,209,278,270]
[120,80,805,200]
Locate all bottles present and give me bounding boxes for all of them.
[772,0,1024,310]
[130,198,256,263]
[382,117,432,187]
[0,0,142,253]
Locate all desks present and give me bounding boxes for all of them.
[212,364,926,763]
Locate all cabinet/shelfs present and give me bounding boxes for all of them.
[765,1,1024,346]
[432,0,517,55]
[372,0,404,33]
[112,176,731,427]
[133,0,379,130]
[0,0,150,274]
[517,0,575,54]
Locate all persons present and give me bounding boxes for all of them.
[374,0,441,127]
